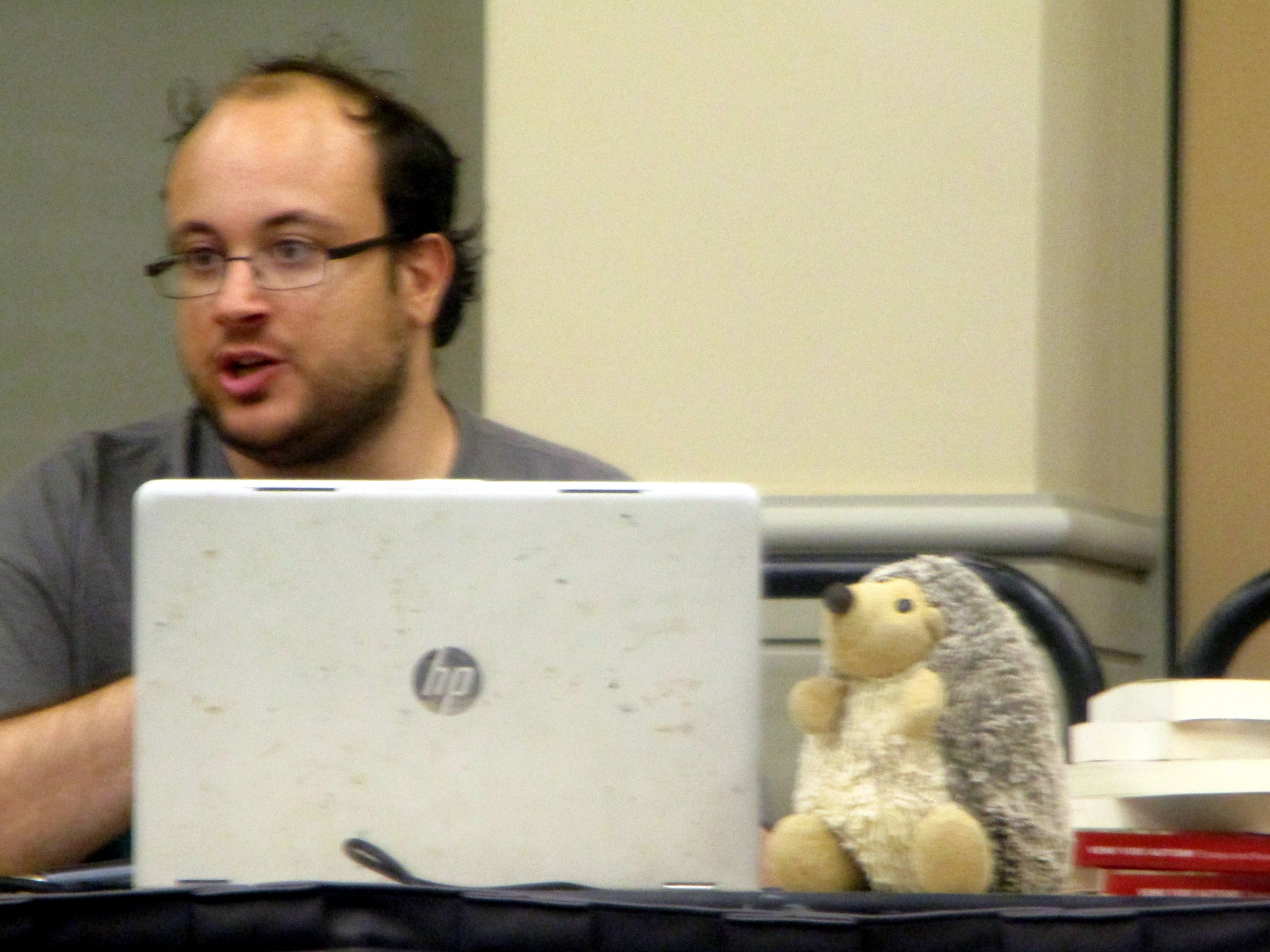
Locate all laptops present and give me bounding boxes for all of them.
[129,474,766,892]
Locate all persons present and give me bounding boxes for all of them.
[0,52,640,880]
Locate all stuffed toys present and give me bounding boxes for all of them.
[762,553,1072,899]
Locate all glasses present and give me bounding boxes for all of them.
[145,233,389,299]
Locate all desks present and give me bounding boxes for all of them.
[0,866,1270,952]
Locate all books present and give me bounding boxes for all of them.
[1054,677,1270,901]
